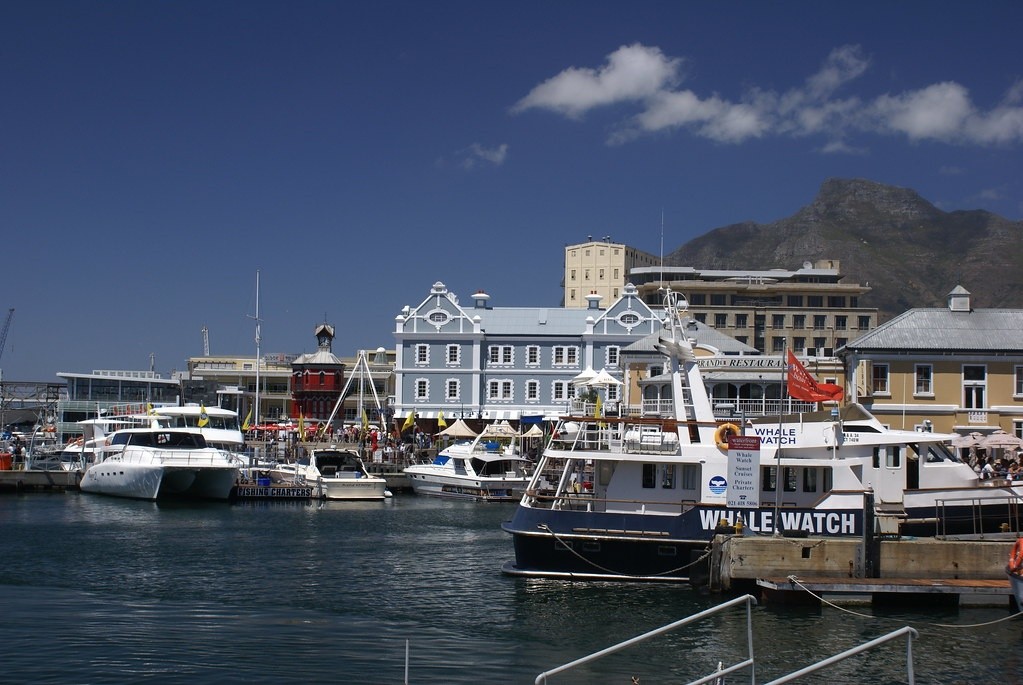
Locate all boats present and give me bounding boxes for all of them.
[291,449,387,499]
[59,402,245,502]
[404,424,533,502]
[501,212,1023,586]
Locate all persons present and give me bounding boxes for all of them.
[974,454,1023,480]
[328,424,397,447]
[7,443,26,470]
[383,443,412,459]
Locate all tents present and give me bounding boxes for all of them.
[480,419,519,443]
[433,419,477,440]
[516,423,544,455]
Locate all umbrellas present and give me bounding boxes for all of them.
[573,364,625,388]
[979,430,1020,456]
[951,431,986,448]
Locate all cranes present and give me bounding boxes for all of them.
[0,308,15,359]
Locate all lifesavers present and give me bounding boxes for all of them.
[714,422,742,450]
[1008,538,1023,572]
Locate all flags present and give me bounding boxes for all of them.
[401,412,414,433]
[197,404,208,428]
[242,412,252,429]
[788,347,844,403]
[550,422,559,438]
[147,403,159,416]
[362,410,368,432]
[594,396,606,428]
[299,412,305,442]
[437,410,447,427]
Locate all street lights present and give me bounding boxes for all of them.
[80,425,86,456]
[30,425,42,450]
